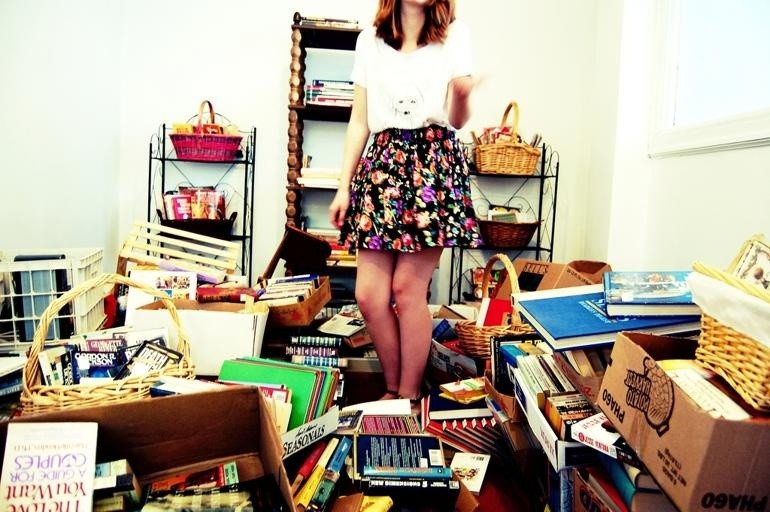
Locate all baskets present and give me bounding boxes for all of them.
[18,273,196,416]
[472,102,540,177]
[692,262,770,411]
[456,253,536,358]
[477,219,538,249]
[169,100,242,161]
[1,248,105,353]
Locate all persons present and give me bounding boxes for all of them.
[329,0,475,418]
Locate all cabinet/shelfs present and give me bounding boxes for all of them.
[287,9,364,280]
[147,121,258,290]
[449,141,561,305]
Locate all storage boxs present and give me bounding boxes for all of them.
[2,382,300,512]
[496,258,614,300]
[595,330,770,511]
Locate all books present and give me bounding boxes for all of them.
[306,78,354,107]
[300,17,359,30]
[1,186,769,511]
